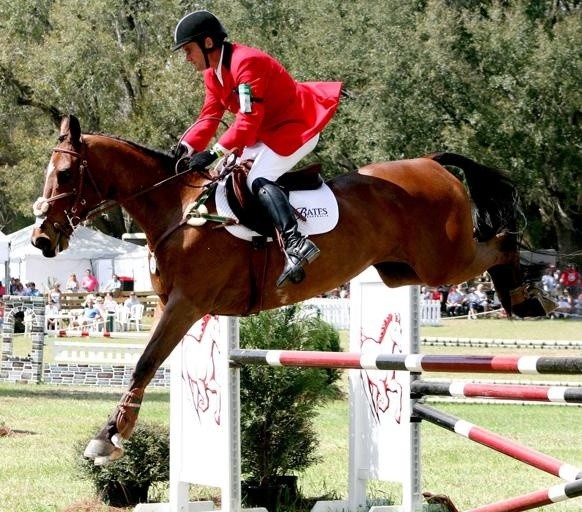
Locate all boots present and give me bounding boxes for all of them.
[259,183,321,289]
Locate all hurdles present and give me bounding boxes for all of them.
[169,271,582,511]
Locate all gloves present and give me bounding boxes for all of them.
[188,146,219,171]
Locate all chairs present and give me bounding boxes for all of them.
[44,297,146,336]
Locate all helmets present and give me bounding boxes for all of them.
[169,11,228,51]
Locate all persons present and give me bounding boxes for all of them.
[0,267,141,329]
[318,283,350,299]
[420,261,581,318]
[170,10,344,288]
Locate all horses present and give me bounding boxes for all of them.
[29,112,560,467]
